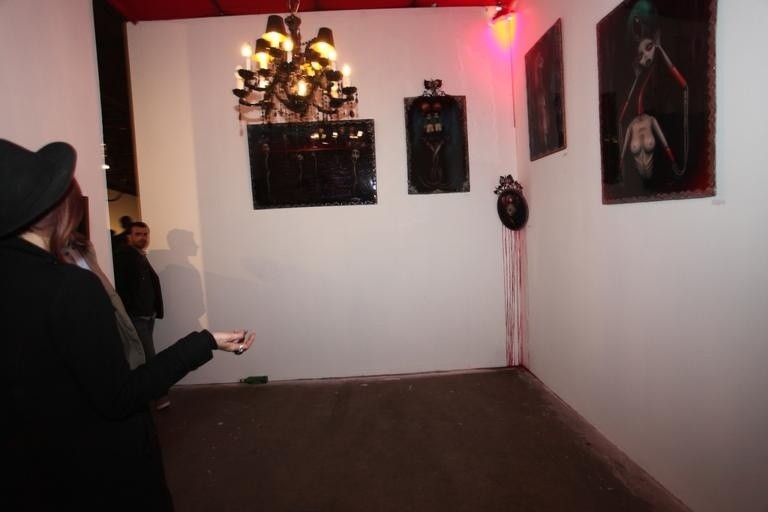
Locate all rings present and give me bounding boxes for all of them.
[239,344,245,353]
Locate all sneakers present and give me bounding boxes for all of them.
[156,398,170,409]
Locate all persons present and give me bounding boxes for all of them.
[116,220,172,412]
[50,177,145,368]
[0,138,256,512]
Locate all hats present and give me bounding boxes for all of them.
[0,138,76,236]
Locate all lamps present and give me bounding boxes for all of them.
[232,0,359,152]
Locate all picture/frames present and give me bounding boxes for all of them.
[244,119,377,210]
[595,0,715,204]
[524,16,567,161]
[403,95,470,195]
